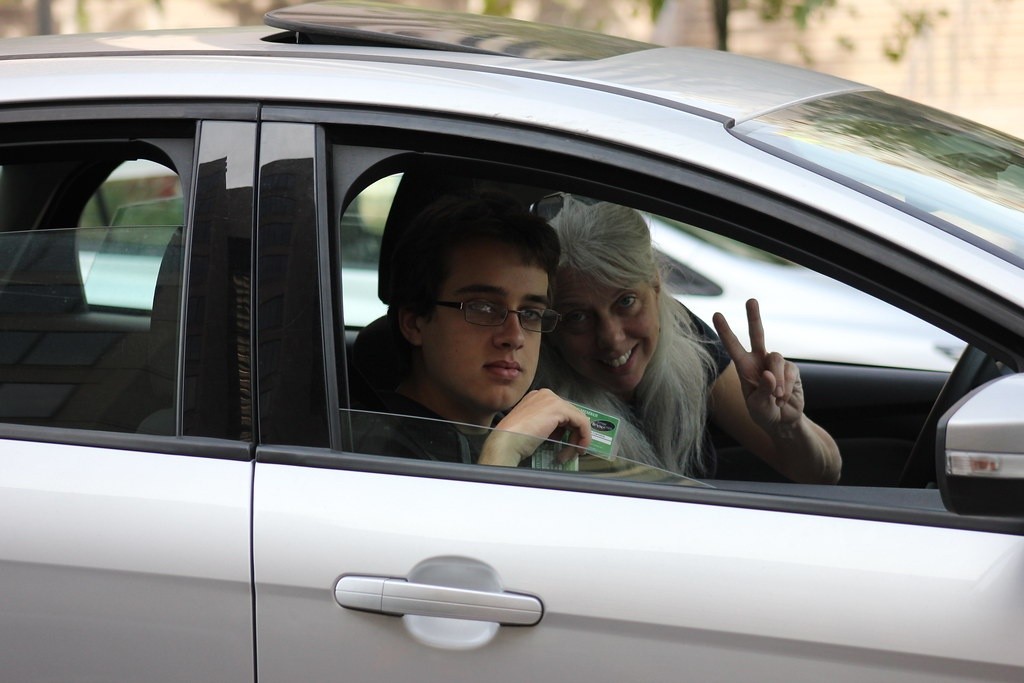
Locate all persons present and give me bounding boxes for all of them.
[331,190,593,470]
[523,192,848,494]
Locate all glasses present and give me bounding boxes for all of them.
[430,297,562,332]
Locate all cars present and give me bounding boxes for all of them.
[0,2,1022,682]
[85,161,969,375]
